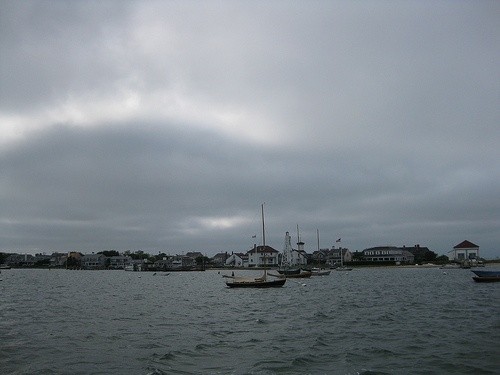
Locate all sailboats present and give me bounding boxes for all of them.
[223,204,353,288]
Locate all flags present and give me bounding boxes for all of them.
[252,235,256,238]
[336,239,341,242]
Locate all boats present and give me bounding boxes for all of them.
[472,276,500,282]
[470,267,500,276]
[0,264,11,270]
